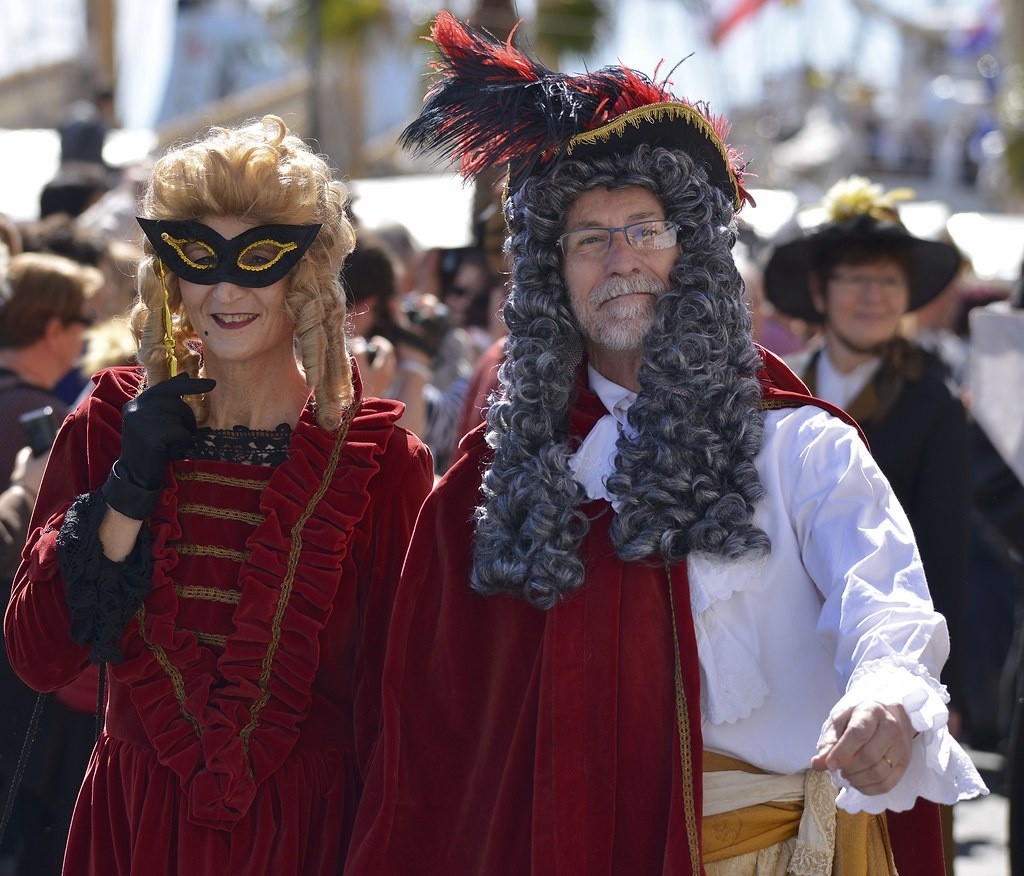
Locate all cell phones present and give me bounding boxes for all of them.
[17,406,58,458]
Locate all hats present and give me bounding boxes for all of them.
[395,9,756,220]
[761,181,962,321]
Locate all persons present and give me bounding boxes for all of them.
[2,109,433,876]
[343,11,989,876]
[0,145,1024,876]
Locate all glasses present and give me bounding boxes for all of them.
[555,218,681,261]
[821,269,910,295]
[73,308,95,328]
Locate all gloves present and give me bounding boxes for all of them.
[100,370,216,520]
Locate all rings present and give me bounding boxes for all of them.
[883,755,894,769]
